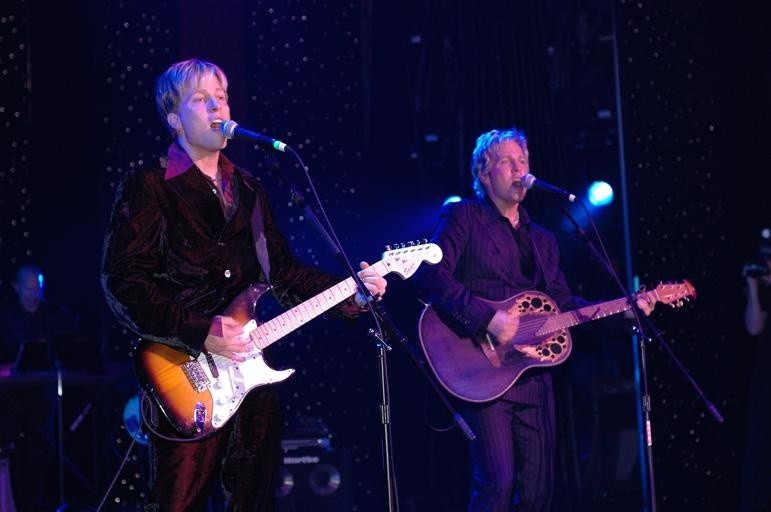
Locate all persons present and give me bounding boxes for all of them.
[740,243,771,511]
[0,266,86,511]
[102,58,388,511]
[414,125,658,512]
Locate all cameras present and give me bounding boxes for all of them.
[746,263,769,278]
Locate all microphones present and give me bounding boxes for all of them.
[521,173,578,204]
[218,120,293,155]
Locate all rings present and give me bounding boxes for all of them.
[371,288,381,296]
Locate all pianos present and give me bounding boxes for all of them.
[0,358,128,389]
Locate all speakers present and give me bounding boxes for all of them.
[275,448,360,512]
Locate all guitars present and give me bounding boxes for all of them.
[418,280,696,402]
[132,238,443,442]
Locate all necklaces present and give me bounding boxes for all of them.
[212,166,221,184]
[512,210,519,229]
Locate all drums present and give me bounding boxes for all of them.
[113,396,152,466]
